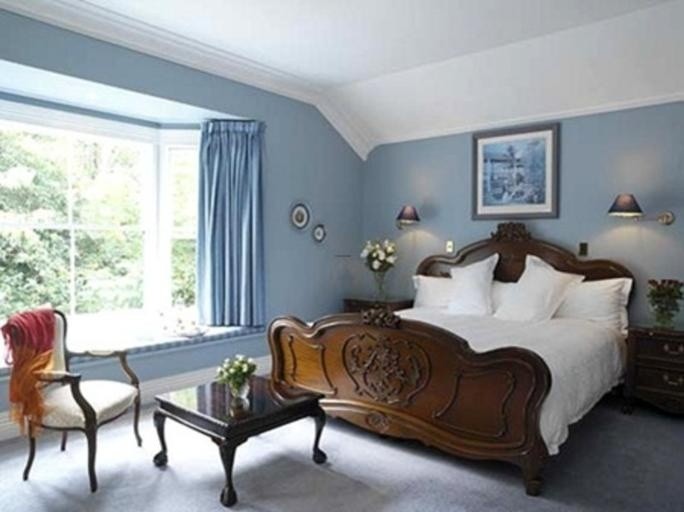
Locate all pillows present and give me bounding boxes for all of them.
[449,253,499,317]
[412,276,502,317]
[500,278,633,339]
[495,253,587,325]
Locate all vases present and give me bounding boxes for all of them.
[370,271,388,301]
[231,379,250,400]
[652,312,674,331]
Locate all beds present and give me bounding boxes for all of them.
[267,224,635,496]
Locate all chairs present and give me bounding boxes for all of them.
[9,309,142,491]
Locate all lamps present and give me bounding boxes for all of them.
[396,205,420,231]
[607,194,674,226]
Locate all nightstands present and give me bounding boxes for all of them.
[343,299,411,312]
[621,327,684,414]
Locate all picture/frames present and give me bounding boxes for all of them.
[470,122,560,220]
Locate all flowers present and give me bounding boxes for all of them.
[360,238,397,273]
[215,355,255,394]
[647,279,684,320]
